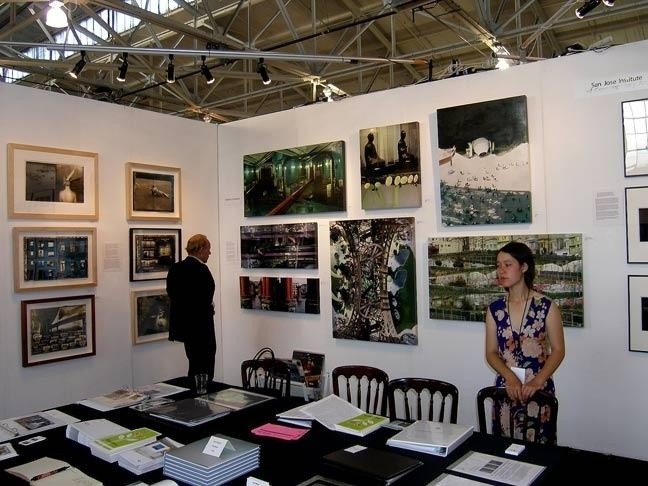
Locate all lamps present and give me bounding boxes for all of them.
[323,88,334,103]
[199,55,215,85]
[256,58,271,85]
[69,51,88,80]
[45,0,69,28]
[166,55,176,83]
[574,2,605,21]
[116,53,129,82]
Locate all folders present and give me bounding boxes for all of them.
[276,402,321,430]
[385,420,474,458]
[321,445,424,486]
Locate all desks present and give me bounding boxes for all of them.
[0,377,648,485]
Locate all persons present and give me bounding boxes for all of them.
[59,180,77,203]
[397,130,418,164]
[166,234,216,381]
[364,132,386,168]
[485,242,565,445]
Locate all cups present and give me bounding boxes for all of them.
[195,374,208,394]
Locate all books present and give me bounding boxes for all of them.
[1,418,260,486]
[79,382,274,427]
[248,390,547,486]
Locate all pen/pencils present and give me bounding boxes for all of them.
[31,466,70,481]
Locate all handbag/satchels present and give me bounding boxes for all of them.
[240,347,305,399]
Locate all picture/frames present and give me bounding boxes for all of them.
[624,186,648,264]
[20,294,96,367]
[627,274,647,353]
[130,285,170,345]
[11,225,98,292]
[6,141,100,222]
[125,161,183,224]
[128,227,182,283]
[620,96,648,179]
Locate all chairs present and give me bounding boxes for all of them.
[332,365,389,419]
[477,385,559,445]
[240,358,292,398]
[388,376,458,425]
